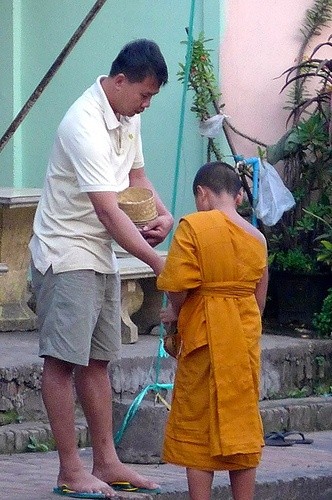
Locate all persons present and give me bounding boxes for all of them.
[28,40,174,498]
[156,161,269,500]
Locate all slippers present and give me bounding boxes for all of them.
[283,431,313,444]
[106,482,160,493]
[263,432,293,446]
[54,484,105,498]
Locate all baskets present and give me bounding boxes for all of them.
[117,187,158,231]
